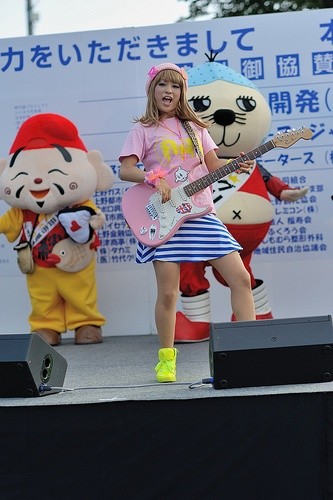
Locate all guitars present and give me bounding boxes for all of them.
[120,126,315,247]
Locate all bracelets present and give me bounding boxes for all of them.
[144,168,165,187]
[226,159,231,164]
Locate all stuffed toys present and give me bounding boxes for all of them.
[0,113,115,347]
[173,50,309,344]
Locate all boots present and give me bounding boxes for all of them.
[155,348,177,382]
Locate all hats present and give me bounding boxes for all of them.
[145,63,188,95]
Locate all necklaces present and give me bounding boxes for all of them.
[163,118,185,145]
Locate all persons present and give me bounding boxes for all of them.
[118,62,256,382]
